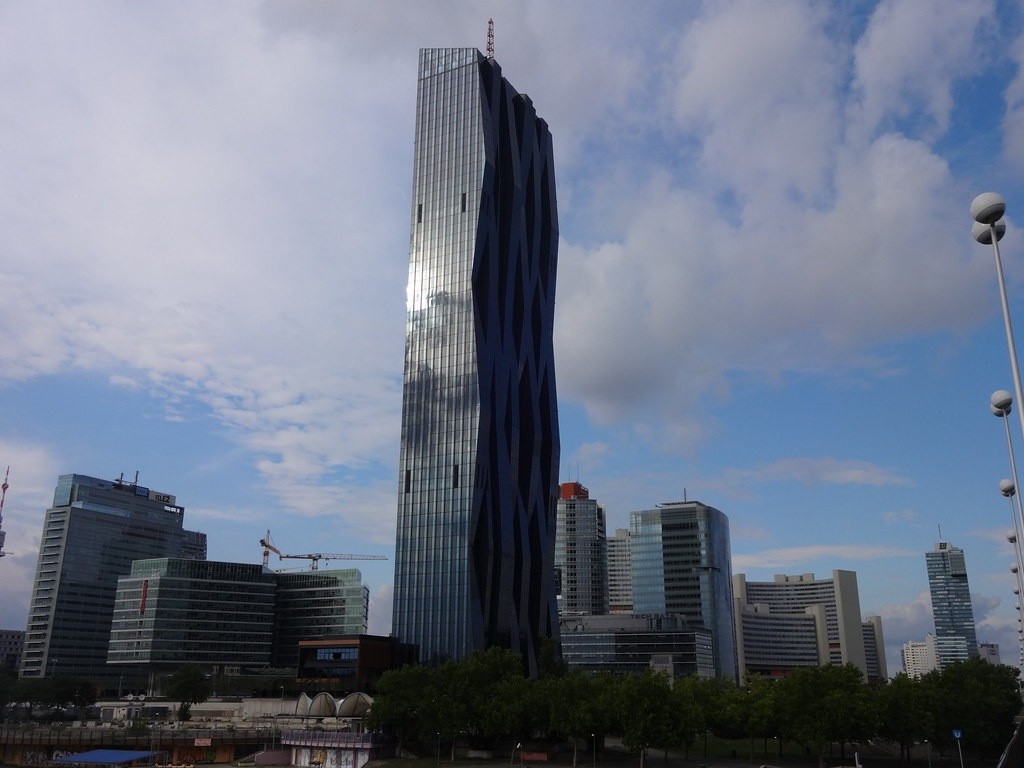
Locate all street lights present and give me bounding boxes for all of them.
[1006,530,1024,608]
[1009,563,1023,625]
[969,191,1023,444]
[996,478,1024,586]
[988,389,1024,539]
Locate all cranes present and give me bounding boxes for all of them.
[259,530,281,567]
[279,550,388,571]
